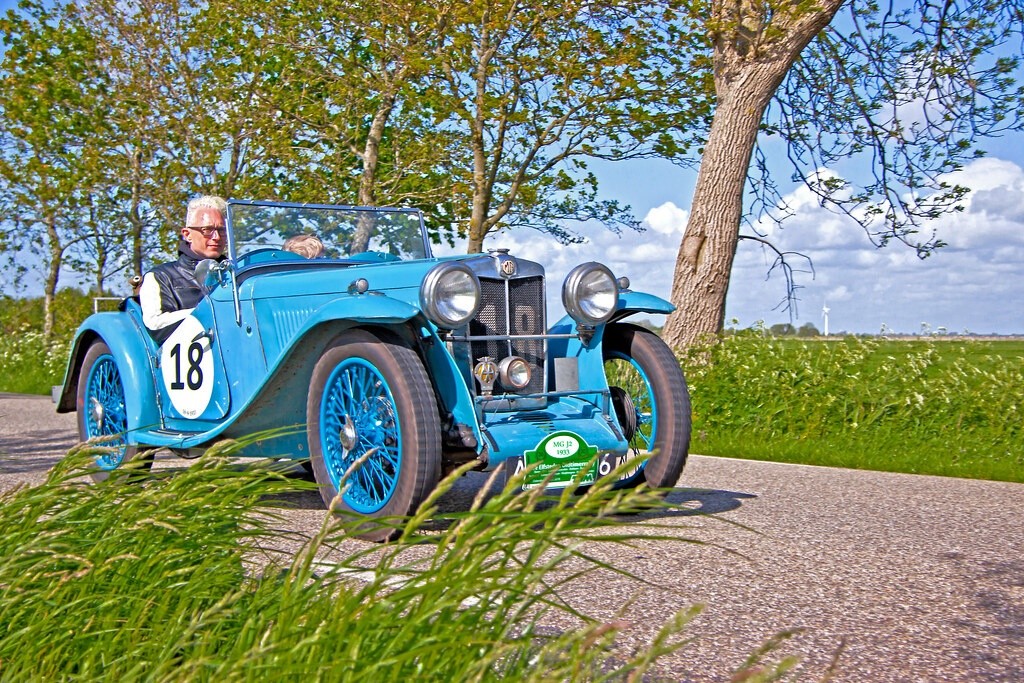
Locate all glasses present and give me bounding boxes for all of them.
[187,226,228,237]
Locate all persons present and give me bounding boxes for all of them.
[140,196,233,346]
[281,235,326,259]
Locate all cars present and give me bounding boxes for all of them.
[49,197,694,546]
